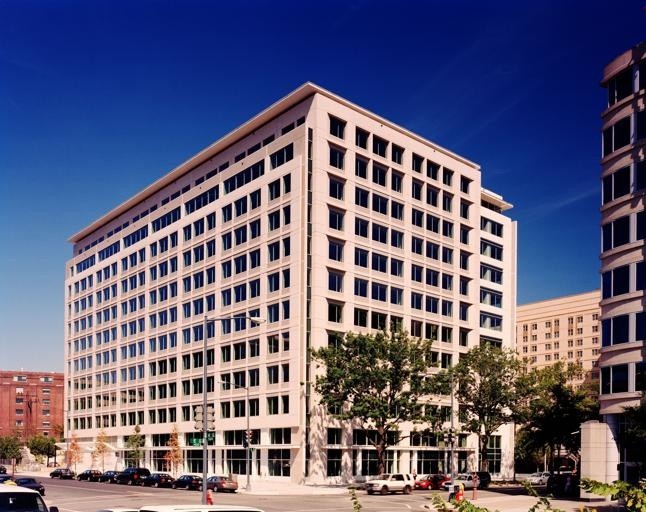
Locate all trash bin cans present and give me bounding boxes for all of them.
[547,473,581,500]
[449,483,465,501]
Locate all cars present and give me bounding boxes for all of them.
[524,471,551,485]
[76,469,238,492]
[0,465,58,512]
[366,472,492,495]
[50,469,75,480]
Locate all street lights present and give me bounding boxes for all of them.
[218,381,252,491]
[202,315,265,506]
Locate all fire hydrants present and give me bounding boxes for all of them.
[473,487,477,500]
[206,489,215,505]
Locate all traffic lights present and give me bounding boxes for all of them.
[195,406,215,431]
[241,429,250,448]
[444,429,454,443]
